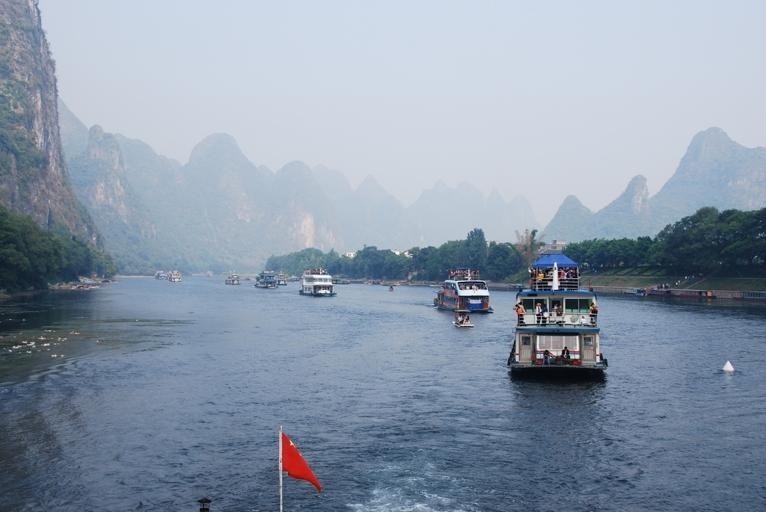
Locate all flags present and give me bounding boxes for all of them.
[282,428,323,491]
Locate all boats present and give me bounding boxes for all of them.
[224,274,240,285]
[623,288,647,297]
[388,285,394,291]
[254,269,288,289]
[154,269,183,282]
[508,252,608,375]
[299,269,353,297]
[432,275,490,328]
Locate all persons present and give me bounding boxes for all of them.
[458,314,463,324]
[464,315,469,323]
[561,346,570,364]
[527,265,580,292]
[515,301,525,327]
[590,303,595,312]
[542,304,548,326]
[535,302,542,325]
[451,267,480,291]
[590,306,599,327]
[557,304,562,326]
